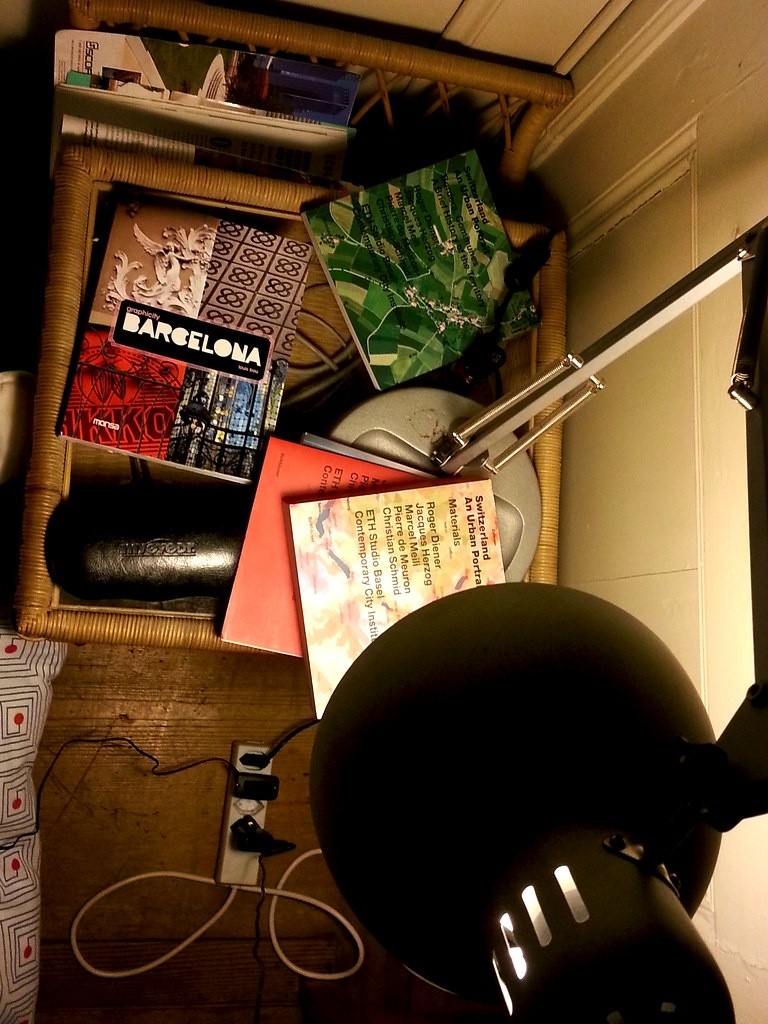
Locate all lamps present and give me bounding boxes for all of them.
[309,217,767,1024]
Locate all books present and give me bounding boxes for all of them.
[216,437,468,658]
[297,143,542,393]
[56,187,313,483]
[44,28,364,193]
[287,477,507,721]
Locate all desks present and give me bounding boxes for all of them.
[16,144,569,651]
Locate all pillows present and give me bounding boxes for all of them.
[0,626,68,1024]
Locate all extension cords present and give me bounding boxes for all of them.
[214,741,274,885]
